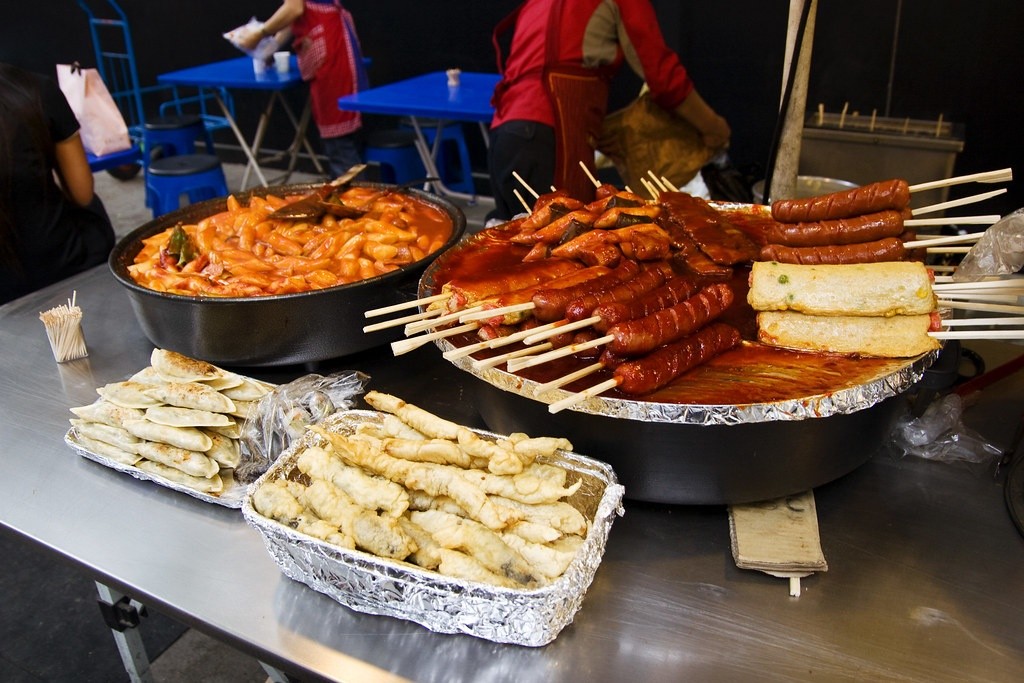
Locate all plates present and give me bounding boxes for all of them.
[61,425,273,507]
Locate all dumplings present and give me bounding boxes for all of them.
[69,348,277,497]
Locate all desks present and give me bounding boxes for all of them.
[158,56,371,193]
[0,264,1024,683]
[338,72,504,226]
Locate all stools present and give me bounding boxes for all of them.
[141,114,213,206]
[145,153,229,221]
[365,130,428,191]
[400,116,476,207]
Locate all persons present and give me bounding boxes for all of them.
[240,0,369,182]
[0,59,114,306]
[486,0,731,222]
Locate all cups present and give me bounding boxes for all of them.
[252,58,267,75]
[273,51,291,73]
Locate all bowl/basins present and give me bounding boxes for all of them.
[750,176,860,207]
[238,409,625,649]
[104,182,468,369]
[410,216,957,505]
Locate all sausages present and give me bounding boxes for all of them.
[534,183,618,211]
[520,258,744,395]
[760,178,941,331]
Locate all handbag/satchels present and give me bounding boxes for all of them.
[54,61,131,156]
